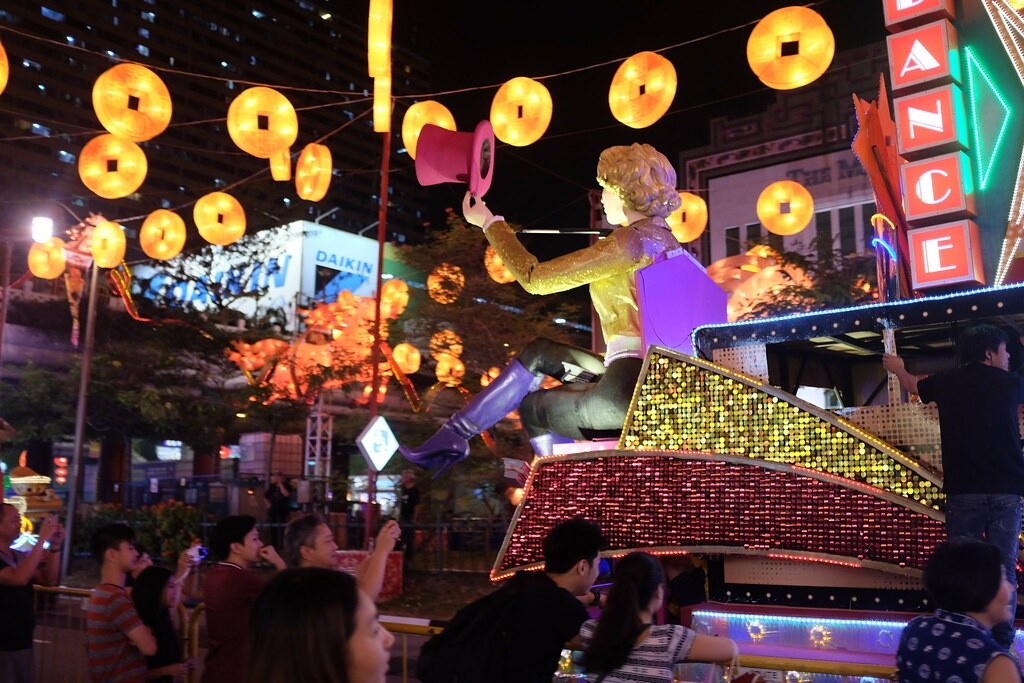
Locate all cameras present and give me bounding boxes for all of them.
[184,545,208,562]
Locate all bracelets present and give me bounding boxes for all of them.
[50,548,60,553]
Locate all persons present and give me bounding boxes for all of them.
[207,515,402,682]
[263,471,296,551]
[399,143,712,480]
[579,552,740,683]
[86,523,205,683]
[0,501,67,682]
[397,470,421,569]
[895,541,1024,682]
[417,519,605,683]
[882,325,1024,652]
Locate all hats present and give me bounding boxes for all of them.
[414,119,494,197]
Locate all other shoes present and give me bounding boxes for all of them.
[991,635,1013,652]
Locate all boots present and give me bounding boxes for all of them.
[528,430,576,456]
[396,360,544,480]
